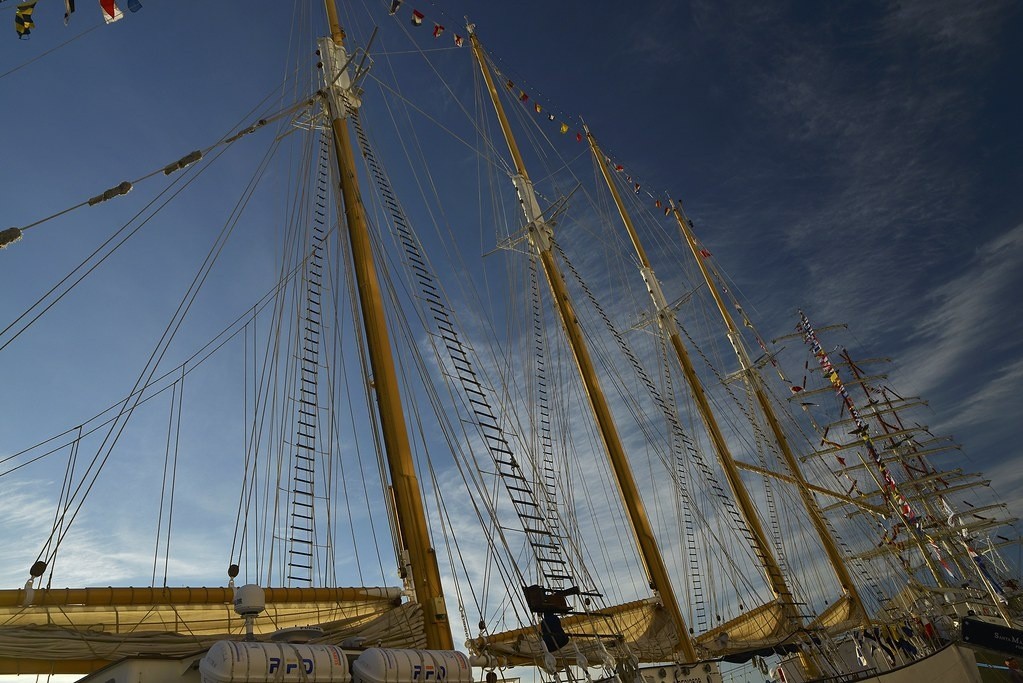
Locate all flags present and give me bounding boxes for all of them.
[691,238,957,581]
[534,104,543,113]
[388,0,404,16]
[547,114,555,121]
[411,9,425,27]
[655,200,671,217]
[961,542,1004,593]
[99,0,124,25]
[627,176,641,192]
[518,91,528,103]
[504,80,514,93]
[64,0,75,25]
[431,24,445,39]
[576,133,582,143]
[605,156,611,166]
[615,166,624,172]
[561,122,569,134]
[453,33,465,47]
[14,0,36,37]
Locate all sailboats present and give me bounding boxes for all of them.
[0,0,1023,683]
